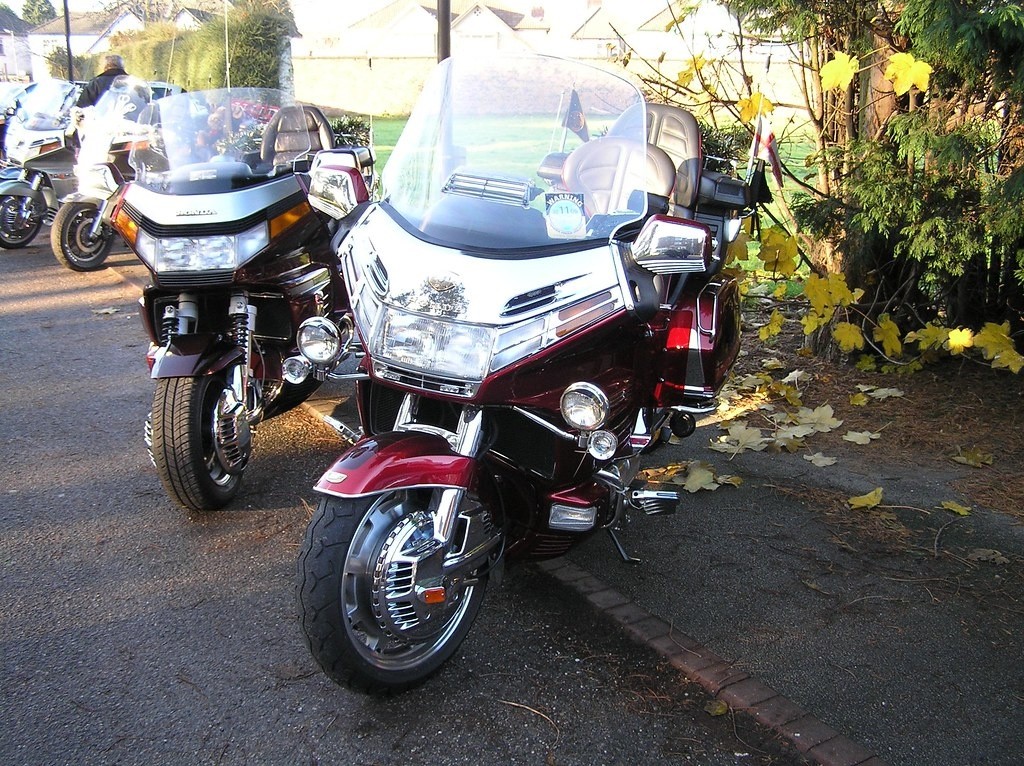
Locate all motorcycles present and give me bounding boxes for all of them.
[102,87,376,516]
[0,77,186,274]
[282,50,770,696]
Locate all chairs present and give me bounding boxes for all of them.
[260,107,333,171]
[560,136,676,227]
[537,104,702,302]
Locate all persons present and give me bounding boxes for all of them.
[77,55,149,124]
[184,105,226,164]
